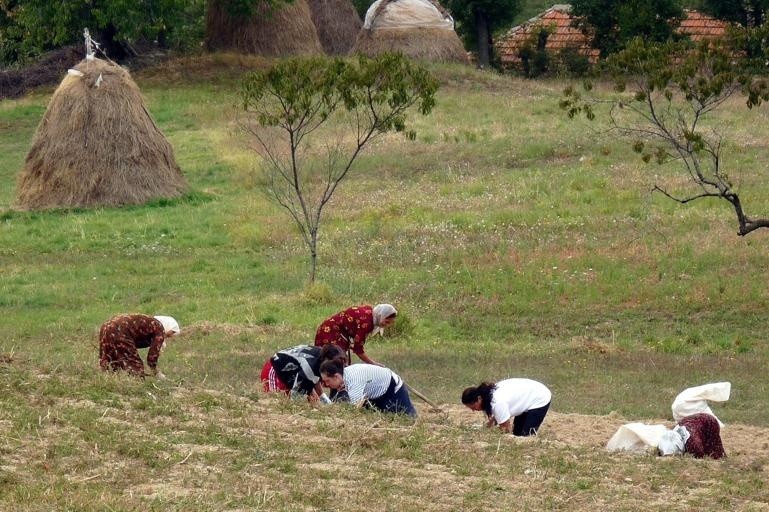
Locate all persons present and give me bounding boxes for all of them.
[98,311,181,382]
[654,411,728,461]
[261,344,348,406]
[315,302,398,401]
[462,376,552,437]
[317,351,418,420]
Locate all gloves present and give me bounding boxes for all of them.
[319,392,332,406]
[154,371,167,383]
